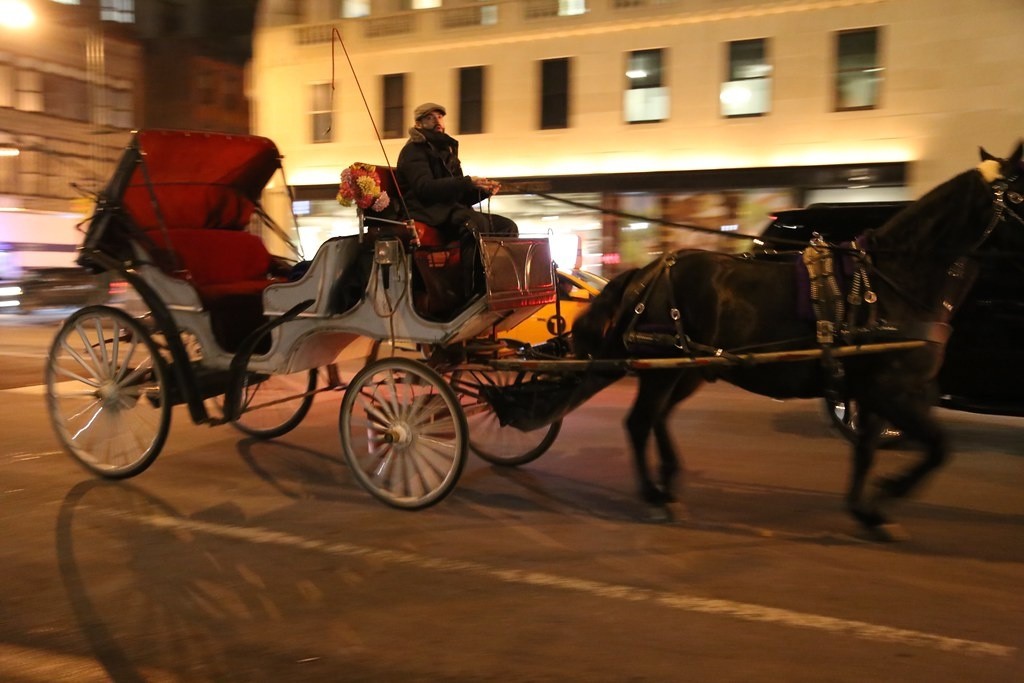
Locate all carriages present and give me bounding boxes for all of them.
[42,128,1024,538]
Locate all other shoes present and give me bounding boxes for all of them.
[459,290,481,312]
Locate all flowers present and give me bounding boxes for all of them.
[335,163,392,216]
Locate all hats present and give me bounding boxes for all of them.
[413,102,446,120]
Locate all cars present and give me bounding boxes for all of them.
[420,256,623,358]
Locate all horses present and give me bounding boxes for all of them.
[569,142,1024,537]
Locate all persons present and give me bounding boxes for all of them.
[395,102,518,301]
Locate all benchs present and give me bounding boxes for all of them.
[350,163,462,271]
[119,120,295,298]
